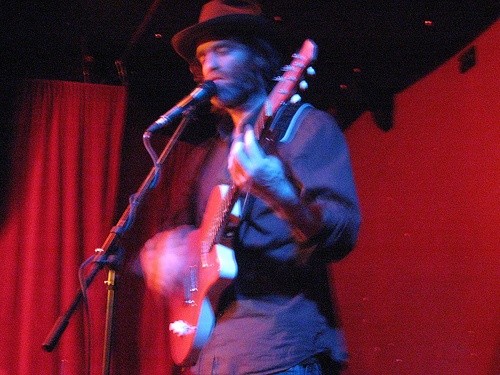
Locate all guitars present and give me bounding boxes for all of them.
[166,38,319,367]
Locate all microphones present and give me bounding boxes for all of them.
[146,79,217,135]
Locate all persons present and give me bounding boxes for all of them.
[137,0,361,375]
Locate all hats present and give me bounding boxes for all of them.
[171,1,292,64]
